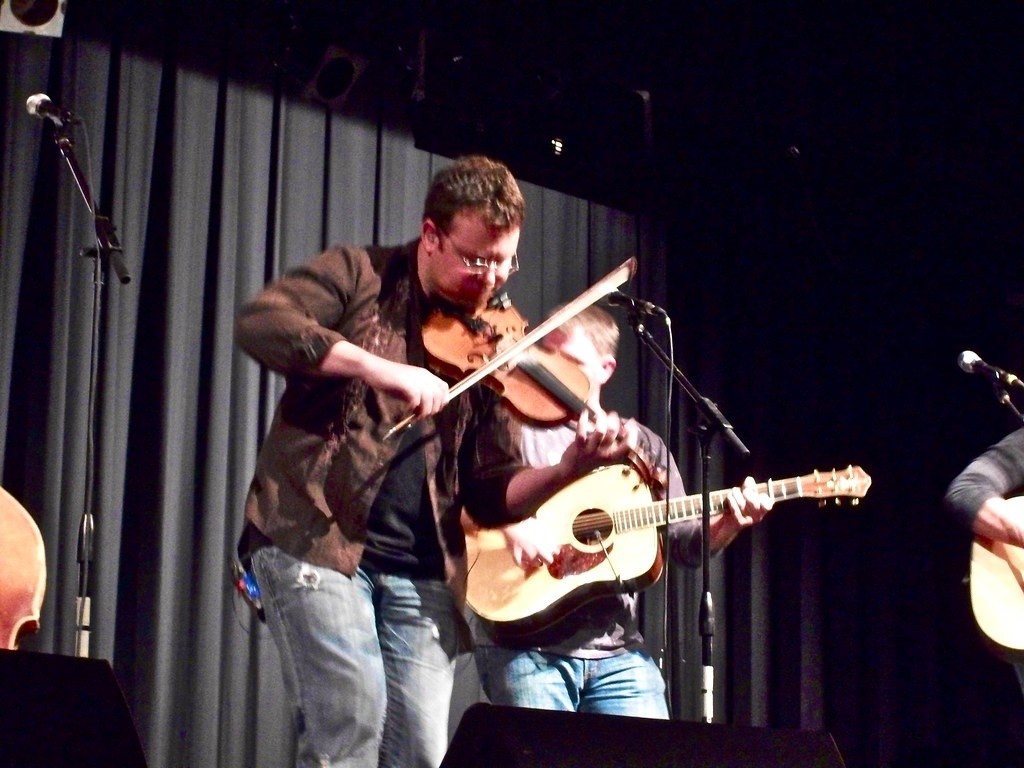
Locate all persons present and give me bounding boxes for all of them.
[943,427,1024,550]
[233,157,638,768]
[469,303,772,720]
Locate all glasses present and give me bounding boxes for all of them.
[442,229,520,277]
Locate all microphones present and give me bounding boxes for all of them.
[956,350,1023,387]
[602,289,667,318]
[26,93,84,125]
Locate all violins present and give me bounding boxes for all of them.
[420,295,675,493]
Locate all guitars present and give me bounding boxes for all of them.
[460,461,875,637]
[970,494,1024,666]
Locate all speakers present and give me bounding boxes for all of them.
[438,704,846,768]
[0,648,150,768]
